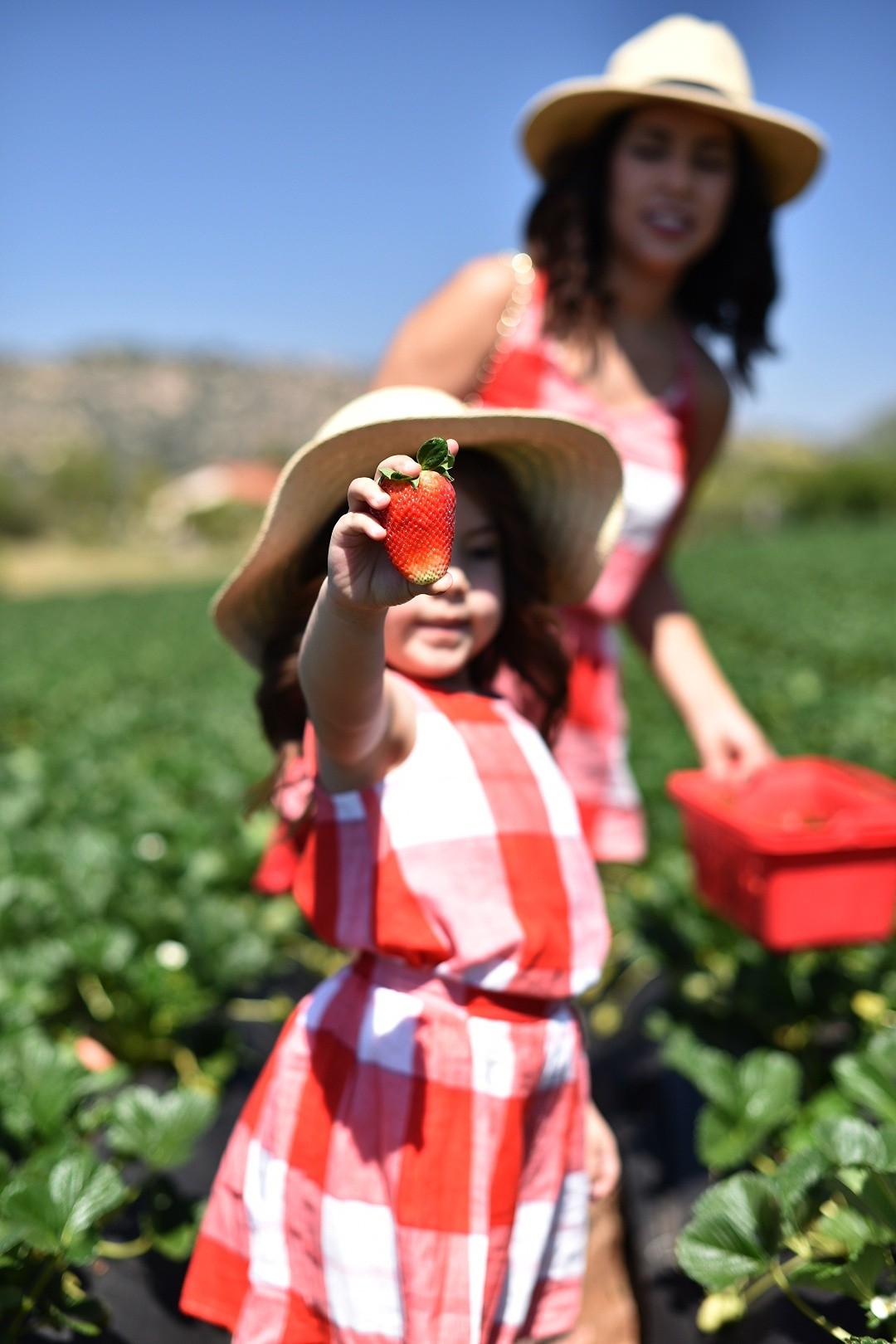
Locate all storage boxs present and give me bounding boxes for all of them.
[662,755,896,952]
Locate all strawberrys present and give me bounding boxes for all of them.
[371,437,458,587]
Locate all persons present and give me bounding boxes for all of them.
[245,13,829,1344]
[178,384,620,1344]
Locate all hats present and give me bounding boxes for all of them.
[519,12,826,212]
[210,387,625,669]
